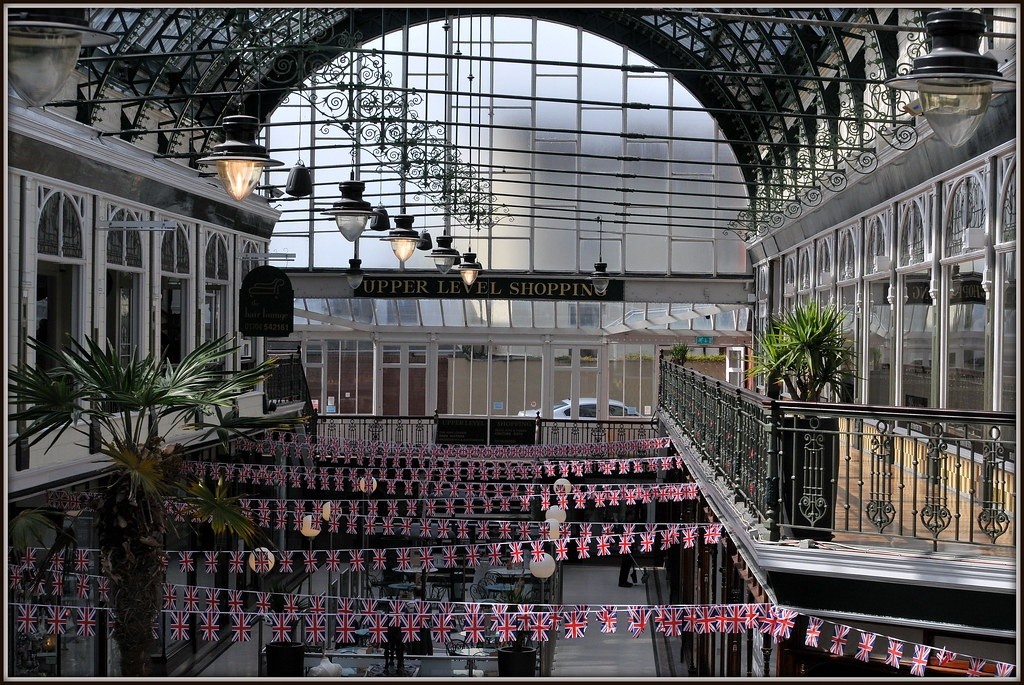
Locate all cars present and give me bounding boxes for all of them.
[517,397,643,420]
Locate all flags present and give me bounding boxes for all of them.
[7,429,1016,677]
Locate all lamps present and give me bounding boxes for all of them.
[319,143,487,288]
[587,219,616,294]
[191,8,286,203]
[283,88,312,203]
[885,11,1020,153]
[6,7,123,106]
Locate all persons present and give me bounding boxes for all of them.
[307,656,343,678]
[618,509,635,587]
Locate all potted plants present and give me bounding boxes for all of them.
[260,584,323,678]
[487,582,557,678]
[741,299,867,541]
